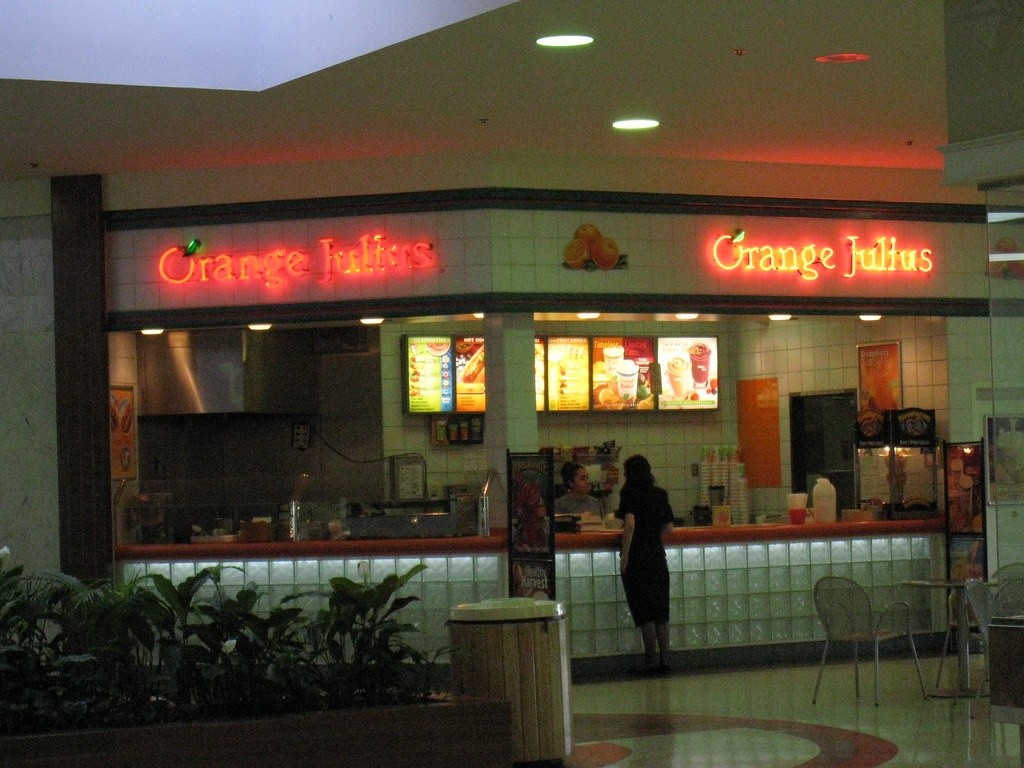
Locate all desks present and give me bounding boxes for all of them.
[901,579,999,698]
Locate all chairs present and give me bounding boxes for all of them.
[812,575,930,703]
[932,561,1023,718]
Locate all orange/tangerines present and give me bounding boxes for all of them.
[564,224,620,270]
[598,388,615,403]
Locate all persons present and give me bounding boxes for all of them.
[616,454,673,673]
[552,460,605,519]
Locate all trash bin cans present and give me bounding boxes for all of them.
[448,595,574,768]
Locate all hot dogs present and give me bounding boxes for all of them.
[462,344,484,383]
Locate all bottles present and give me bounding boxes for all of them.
[813,478,836,524]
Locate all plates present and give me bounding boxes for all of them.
[425,343,450,356]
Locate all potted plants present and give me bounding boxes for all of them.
[0,546,515,768]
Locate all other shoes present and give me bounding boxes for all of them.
[640,664,673,678]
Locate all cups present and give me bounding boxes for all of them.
[689,344,711,390]
[789,510,806,524]
[616,360,639,404]
[634,357,653,399]
[603,347,624,391]
[699,445,750,526]
[667,357,691,398]
